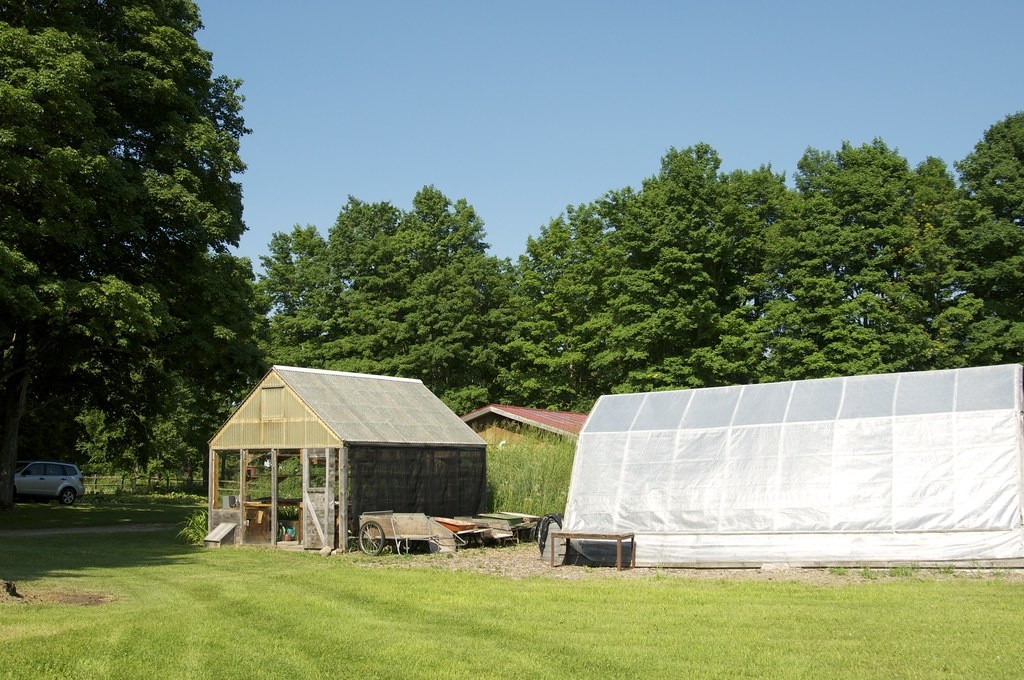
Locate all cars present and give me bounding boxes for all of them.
[15,461,85,506]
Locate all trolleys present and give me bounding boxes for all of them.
[360,509,467,556]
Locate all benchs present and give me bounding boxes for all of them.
[550,530,636,571]
